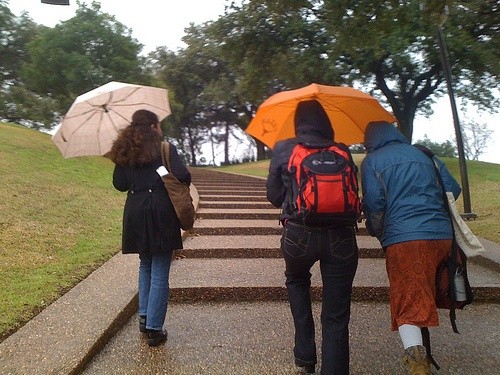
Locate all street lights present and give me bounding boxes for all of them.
[436,4,477,221]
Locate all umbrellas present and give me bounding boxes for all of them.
[51,82,172,159]
[246,84,399,150]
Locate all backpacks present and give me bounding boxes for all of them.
[286,137,362,224]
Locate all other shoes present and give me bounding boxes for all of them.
[148,329,167,346]
[293,356,316,373]
[402,344,432,375]
[139,317,146,332]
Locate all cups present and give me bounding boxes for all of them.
[454,266,467,301]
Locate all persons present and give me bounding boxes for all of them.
[265,99,360,375]
[111,109,192,347]
[358,121,461,375]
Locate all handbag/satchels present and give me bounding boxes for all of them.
[434,242,472,309]
[159,141,195,230]
